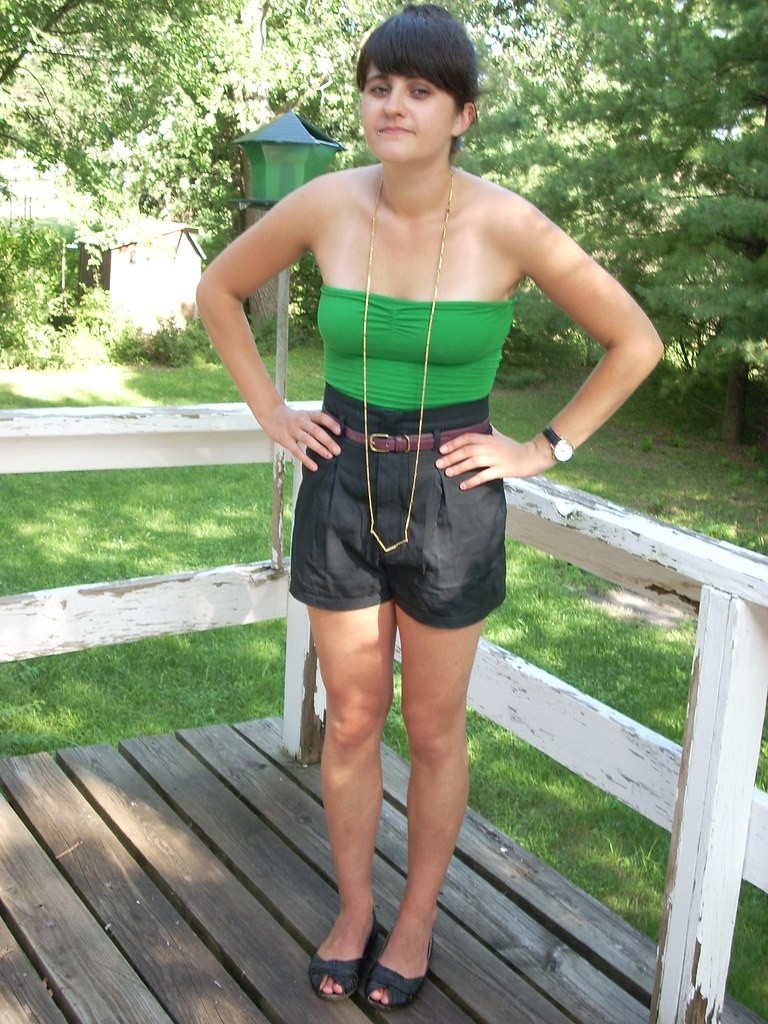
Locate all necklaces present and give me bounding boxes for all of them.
[363,170,455,552]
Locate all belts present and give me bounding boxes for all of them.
[320,405,490,453]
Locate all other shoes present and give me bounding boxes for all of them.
[364,925,435,1010]
[308,909,378,1001]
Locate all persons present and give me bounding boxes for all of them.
[196,4,666,1011]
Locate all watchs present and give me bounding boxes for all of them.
[541,425,575,464]
